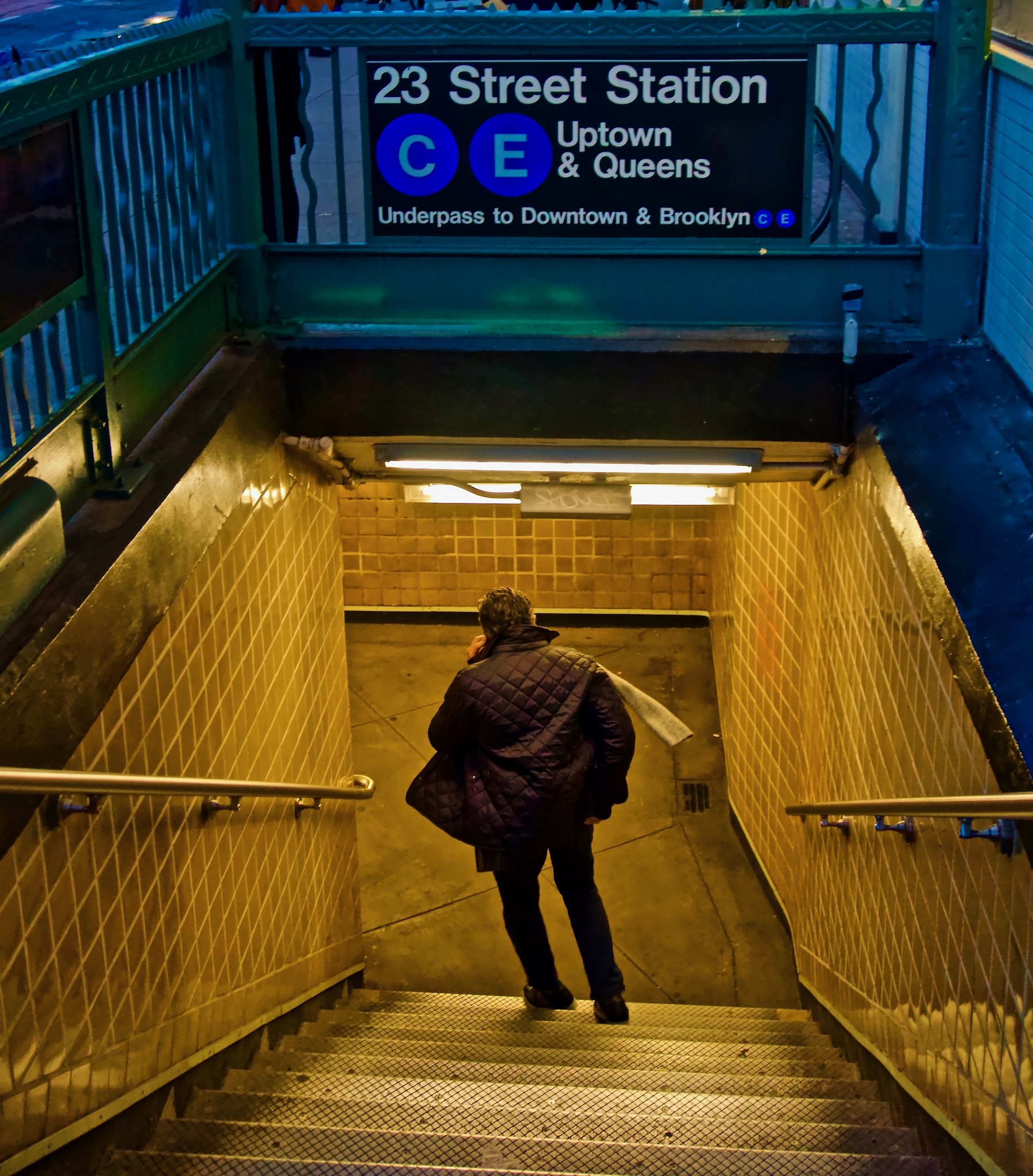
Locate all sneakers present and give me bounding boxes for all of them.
[523,985,578,1010]
[595,996,629,1023]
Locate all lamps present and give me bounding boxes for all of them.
[374,443,763,473]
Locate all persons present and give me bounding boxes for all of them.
[173,0,809,243]
[404,587,694,1025]
